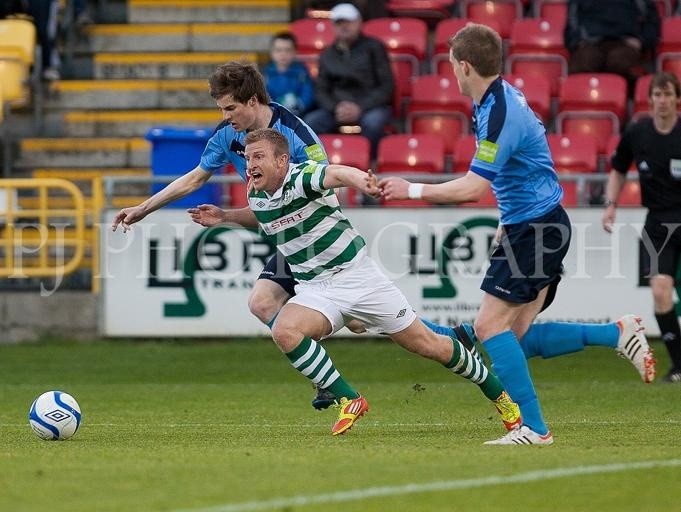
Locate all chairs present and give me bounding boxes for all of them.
[0,0,64,178]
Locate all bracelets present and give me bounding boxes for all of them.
[408,182,424,202]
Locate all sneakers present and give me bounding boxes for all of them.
[329,392,368,436]
[614,315,656,383]
[663,368,681,383]
[312,386,340,410]
[452,323,486,366]
[483,425,554,445]
[492,391,523,431]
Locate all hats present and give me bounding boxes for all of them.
[329,4,359,24]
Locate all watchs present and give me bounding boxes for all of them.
[604,199,616,207]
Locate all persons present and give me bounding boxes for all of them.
[601,71,681,383]
[302,2,396,158]
[186,126,523,436]
[110,61,486,411]
[562,2,663,99]
[256,29,313,116]
[377,22,659,445]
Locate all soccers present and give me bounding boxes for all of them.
[28,391,81,440]
